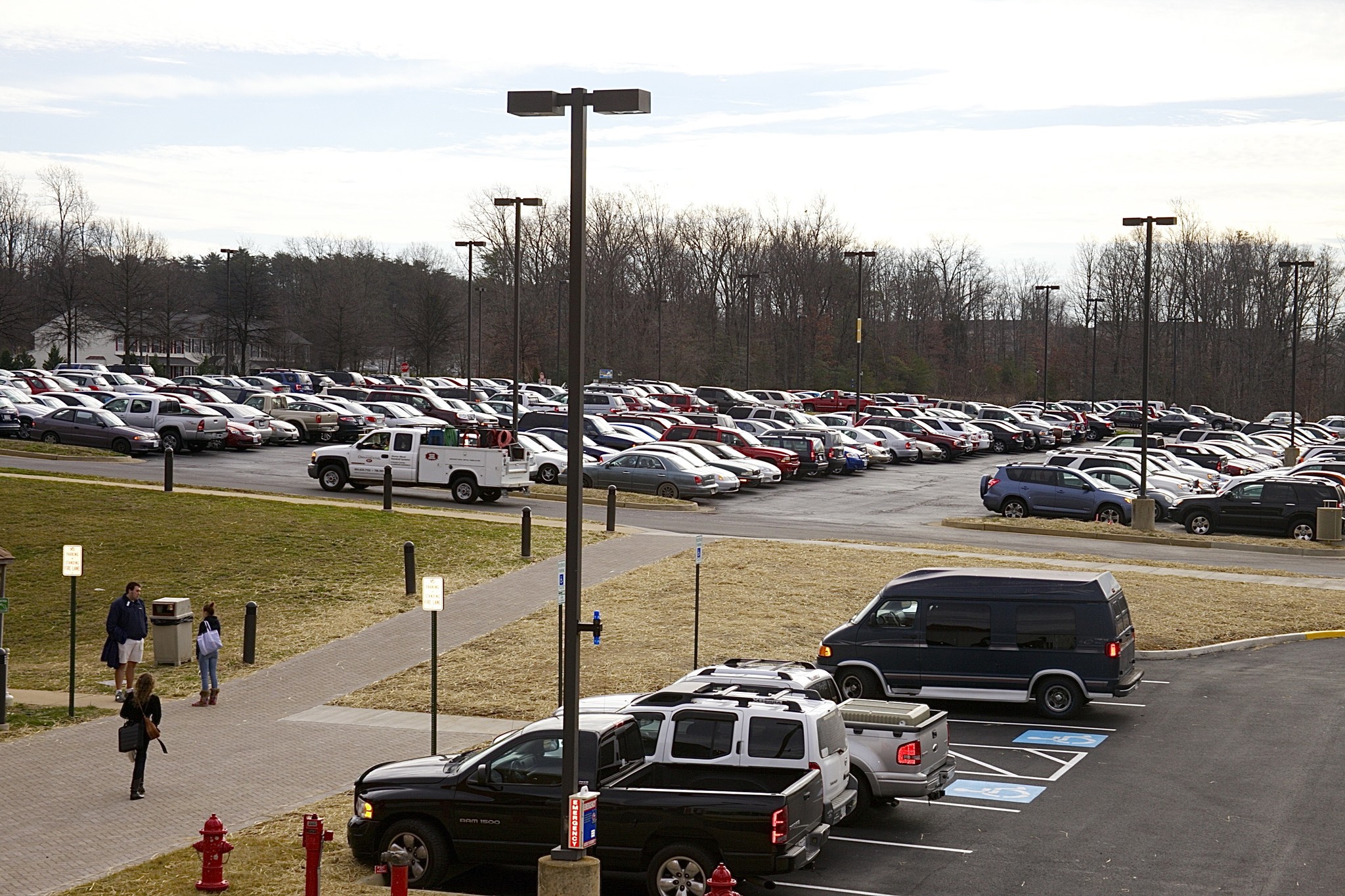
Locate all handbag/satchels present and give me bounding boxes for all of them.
[197,621,223,656]
[119,721,140,753]
[144,718,160,740]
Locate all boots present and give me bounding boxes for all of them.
[192,690,209,706]
[209,688,220,705]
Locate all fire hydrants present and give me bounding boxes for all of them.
[704,863,740,895]
[380,843,410,896]
[192,813,234,891]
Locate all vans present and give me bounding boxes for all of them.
[812,566,1145,722]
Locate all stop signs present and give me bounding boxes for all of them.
[400,362,410,372]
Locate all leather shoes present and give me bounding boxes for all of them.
[130,791,144,800]
[137,788,145,793]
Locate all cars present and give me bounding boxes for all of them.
[0,361,1345,542]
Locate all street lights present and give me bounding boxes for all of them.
[346,711,832,896]
[1035,285,1060,412]
[474,287,487,378]
[738,273,760,391]
[1122,215,1177,528]
[455,240,486,402]
[844,250,878,422]
[1167,317,1183,404]
[1278,259,1316,468]
[221,248,239,385]
[557,279,570,386]
[493,197,543,443]
[505,85,653,860]
[1086,297,1105,414]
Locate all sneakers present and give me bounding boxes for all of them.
[115,692,125,702]
[124,692,130,698]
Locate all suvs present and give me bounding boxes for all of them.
[609,680,860,828]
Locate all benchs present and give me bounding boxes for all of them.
[54,415,74,420]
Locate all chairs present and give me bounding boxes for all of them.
[310,406,317,411]
[641,458,647,468]
[647,459,656,469]
[89,417,96,426]
[133,404,142,411]
[561,434,567,446]
[299,406,308,410]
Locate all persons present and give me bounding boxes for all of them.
[120,673,162,800]
[372,437,390,450]
[1081,411,1088,442]
[192,602,221,706]
[106,582,148,703]
[1298,420,1305,427]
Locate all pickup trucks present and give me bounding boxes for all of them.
[555,659,957,830]
[306,423,537,505]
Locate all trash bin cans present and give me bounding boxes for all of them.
[1316,507,1343,540]
[149,597,194,666]
